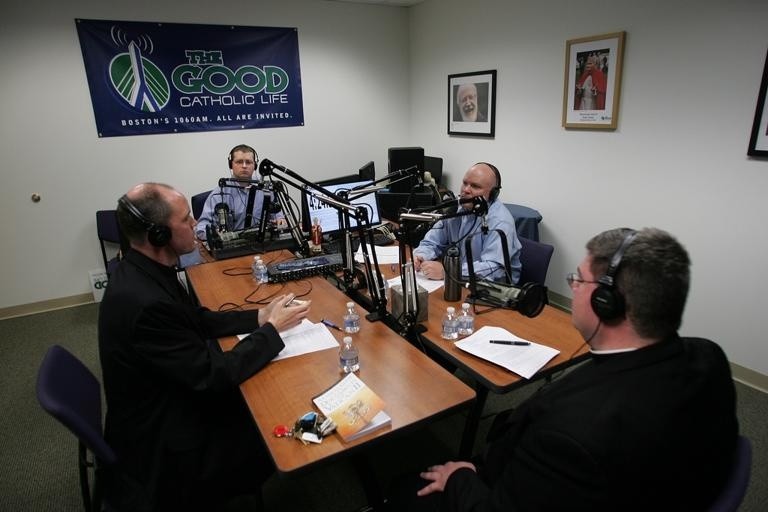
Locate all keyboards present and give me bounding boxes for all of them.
[322,235,394,255]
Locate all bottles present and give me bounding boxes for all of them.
[443,245,462,302]
[256,261,269,283]
[459,303,475,336]
[344,302,361,334]
[337,336,360,373]
[441,306,459,339]
[250,255,262,277]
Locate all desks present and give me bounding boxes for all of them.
[184,247,478,473]
[309,246,595,411]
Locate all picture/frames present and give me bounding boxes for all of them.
[562,30,626,130]
[748,48,768,159]
[446,69,496,138]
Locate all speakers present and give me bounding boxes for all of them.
[387,146,425,193]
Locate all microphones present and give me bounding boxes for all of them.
[206,202,278,250]
[476,280,548,318]
[431,190,457,215]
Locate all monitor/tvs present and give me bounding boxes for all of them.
[305,179,381,238]
[302,174,362,233]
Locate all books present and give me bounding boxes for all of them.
[312,370,392,443]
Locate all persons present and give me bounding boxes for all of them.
[455,84,486,122]
[416,228,737,512]
[410,163,523,286]
[196,144,288,242]
[574,49,610,111]
[99,180,312,512]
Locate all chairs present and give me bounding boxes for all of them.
[516,236,555,290]
[713,436,753,512]
[97,209,124,281]
[191,189,214,222]
[35,345,119,512]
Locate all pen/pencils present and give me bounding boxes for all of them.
[321,319,342,331]
[489,340,531,346]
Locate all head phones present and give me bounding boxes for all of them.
[590,232,639,326]
[228,145,259,172]
[474,162,501,203]
[120,194,172,249]
